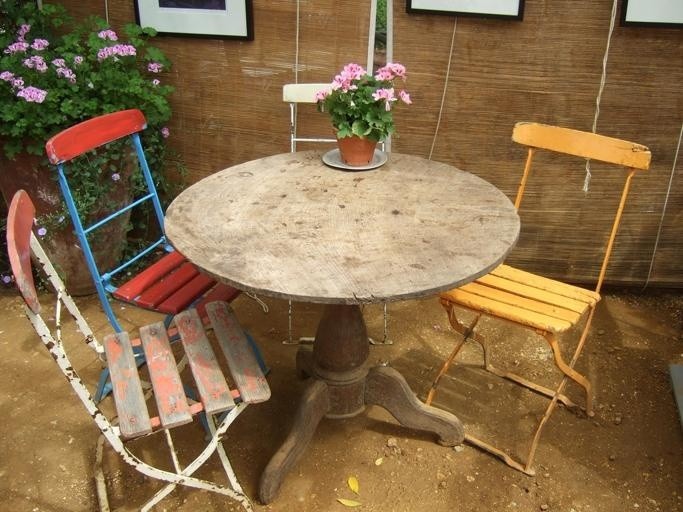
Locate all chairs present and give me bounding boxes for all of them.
[45,109,276,445]
[8,190,270,511]
[283,82,394,344]
[421,123,651,476]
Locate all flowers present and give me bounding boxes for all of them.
[315,63,410,143]
[0,0,187,290]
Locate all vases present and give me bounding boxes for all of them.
[1,137,138,298]
[335,125,377,165]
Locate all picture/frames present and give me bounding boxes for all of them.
[619,0,683,29]
[135,0,253,41]
[407,0,524,20]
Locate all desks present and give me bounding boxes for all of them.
[166,149,520,505]
